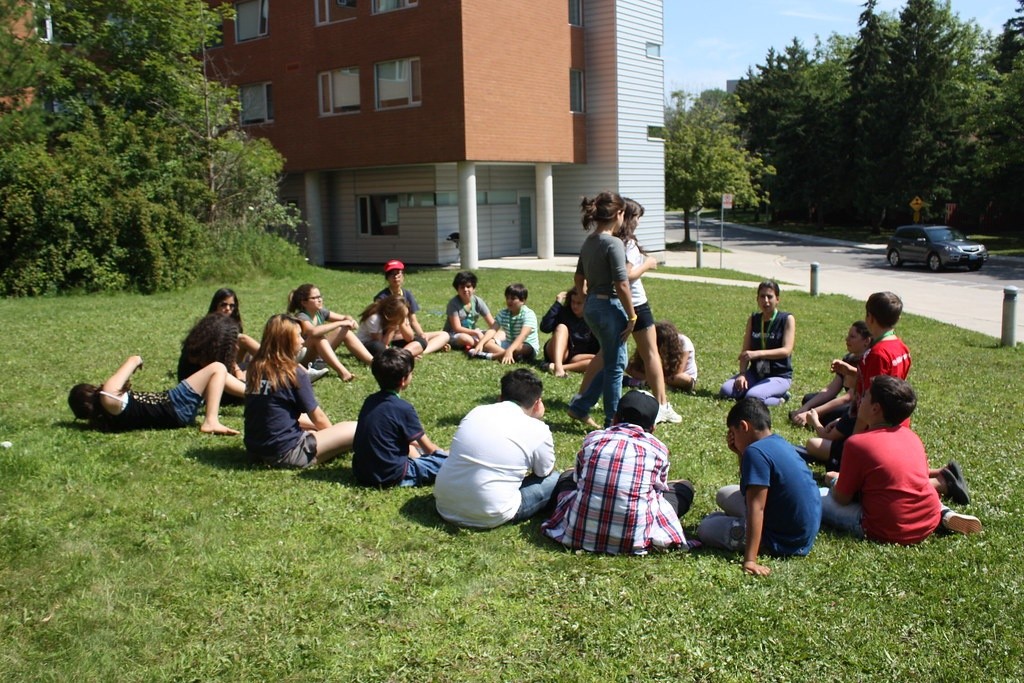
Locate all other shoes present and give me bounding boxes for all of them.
[940,462,969,505]
[542,362,555,373]
[942,512,982,535]
[469,347,487,359]
[441,343,451,352]
[306,363,329,384]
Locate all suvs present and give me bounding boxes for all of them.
[886,225,989,272]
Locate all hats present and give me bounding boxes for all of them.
[384,261,404,272]
[617,389,659,431]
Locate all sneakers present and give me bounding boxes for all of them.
[657,401,682,424]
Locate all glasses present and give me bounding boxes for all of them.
[220,301,237,310]
[309,294,325,302]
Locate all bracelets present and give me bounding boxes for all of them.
[628,314,637,321]
[233,365,240,373]
[830,477,838,486]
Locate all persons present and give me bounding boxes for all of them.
[566,191,636,428]
[434,369,561,531]
[177,289,328,405]
[356,295,427,360]
[612,198,681,424]
[626,321,697,395]
[817,375,982,545]
[244,315,358,468]
[720,280,795,405]
[67,356,246,435]
[697,396,823,574]
[352,347,448,490]
[469,282,540,364]
[540,389,700,556]
[373,260,451,356]
[789,291,969,504]
[285,283,375,381]
[539,284,601,379]
[443,271,495,349]
